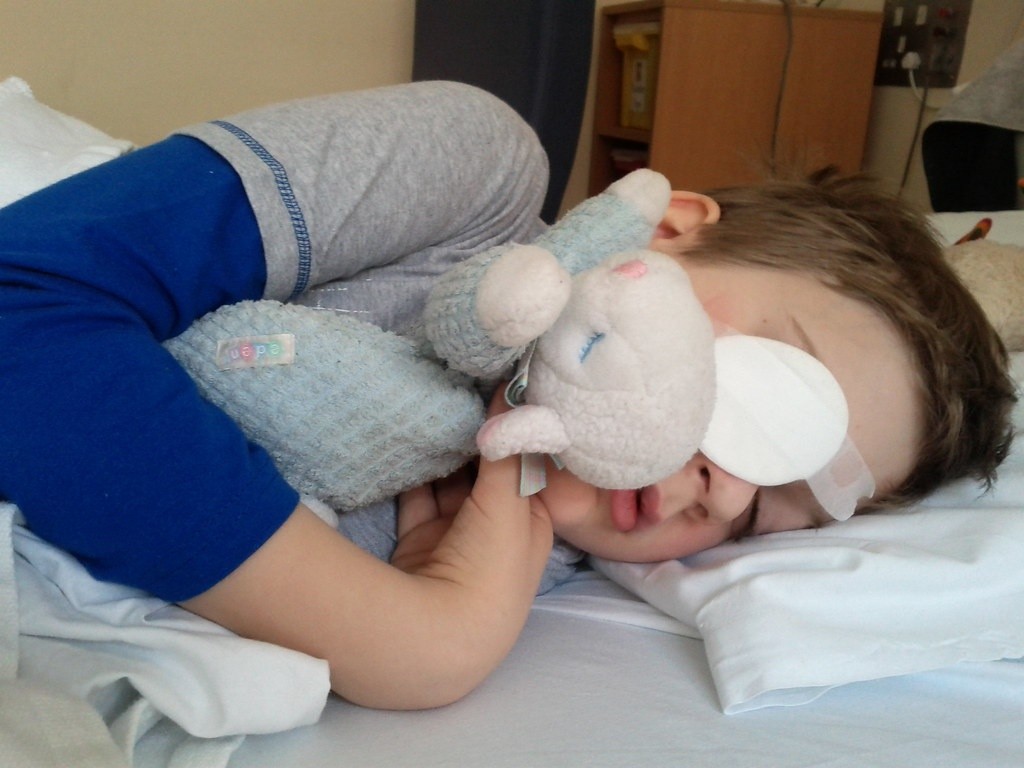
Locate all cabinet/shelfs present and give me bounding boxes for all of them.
[586,0,887,197]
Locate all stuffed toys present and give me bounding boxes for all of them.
[158,170,717,514]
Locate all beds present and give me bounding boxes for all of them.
[0,73,1023,767]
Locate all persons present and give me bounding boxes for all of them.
[921,36,1024,213]
[0,81,1020,711]
